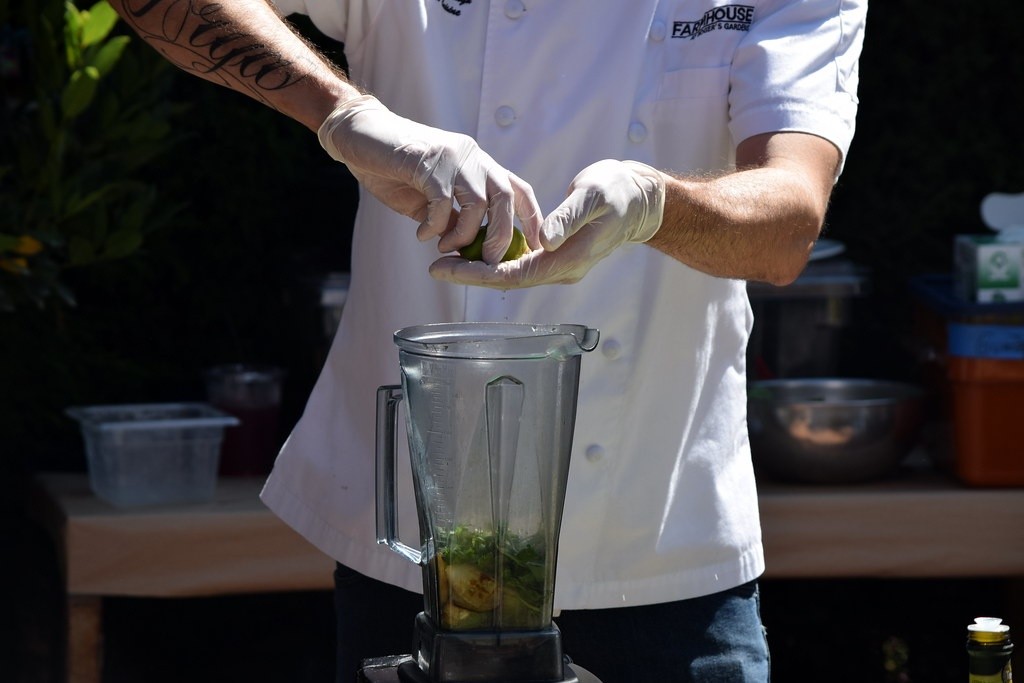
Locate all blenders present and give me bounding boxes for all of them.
[361,321,606,683]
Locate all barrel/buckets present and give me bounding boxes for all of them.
[945,355,1024,488]
[948,315,1024,359]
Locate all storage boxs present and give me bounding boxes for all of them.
[65,402,240,510]
[917,301,1024,489]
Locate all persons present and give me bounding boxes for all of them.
[104,0,867,683]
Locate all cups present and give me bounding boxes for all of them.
[210,367,285,478]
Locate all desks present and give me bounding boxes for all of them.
[29,466,1024,683]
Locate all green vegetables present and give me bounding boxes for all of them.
[436,525,551,599]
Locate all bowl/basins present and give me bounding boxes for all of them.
[748,378,921,481]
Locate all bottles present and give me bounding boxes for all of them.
[966,617,1014,683]
